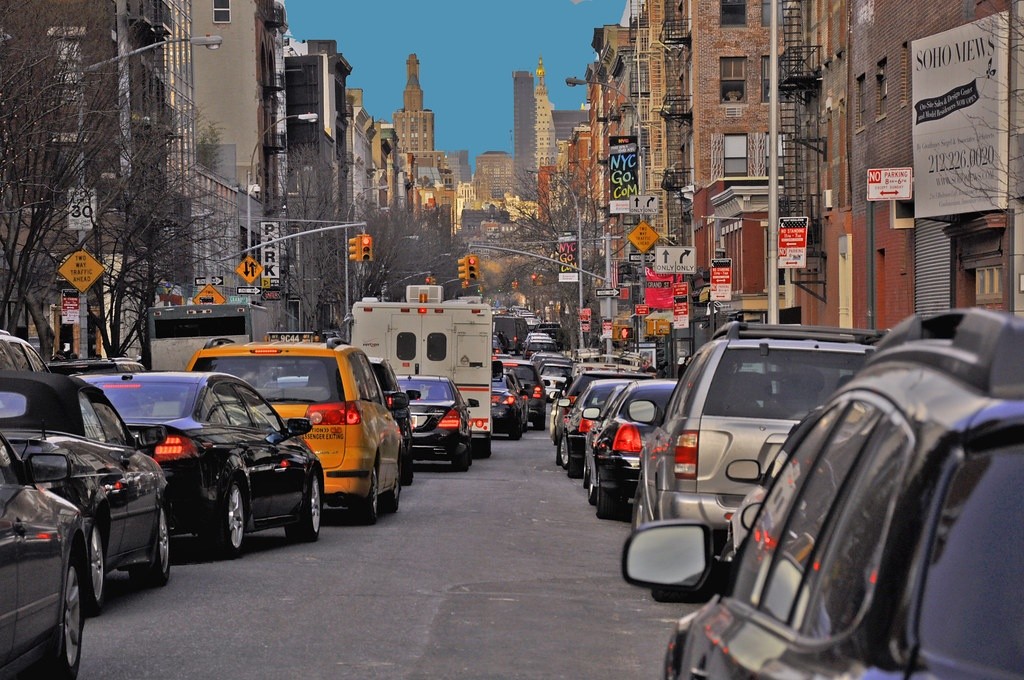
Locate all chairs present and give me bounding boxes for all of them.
[597,393,609,403]
[719,372,788,419]
[307,367,331,394]
[787,371,826,414]
[426,386,446,399]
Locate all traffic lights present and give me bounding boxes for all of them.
[621,327,635,340]
[464,254,480,281]
[361,236,374,262]
[426,277,432,285]
[457,257,467,281]
[349,234,361,262]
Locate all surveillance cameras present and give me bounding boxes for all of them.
[254,186,260,193]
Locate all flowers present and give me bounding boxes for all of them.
[726,91,742,98]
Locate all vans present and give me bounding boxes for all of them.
[340,285,504,459]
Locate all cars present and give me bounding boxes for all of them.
[0,328,326,618]
[490,305,658,478]
[0,429,90,680]
[395,374,479,472]
[582,378,680,521]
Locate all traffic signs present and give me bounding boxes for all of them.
[194,276,225,287]
[594,288,621,297]
[629,252,656,263]
[236,286,262,295]
[866,167,913,201]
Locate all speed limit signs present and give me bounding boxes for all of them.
[67,187,98,231]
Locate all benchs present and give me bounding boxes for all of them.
[255,386,330,402]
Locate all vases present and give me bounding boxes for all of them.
[730,97,737,101]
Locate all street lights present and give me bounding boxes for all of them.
[78,34,224,360]
[247,111,319,344]
[526,168,585,348]
[565,76,646,344]
[344,184,390,343]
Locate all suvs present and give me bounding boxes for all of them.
[625,318,893,560]
[263,357,422,486]
[620,303,1024,680]
[186,336,411,526]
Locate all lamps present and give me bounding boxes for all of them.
[702,322,710,329]
[876,69,883,77]
